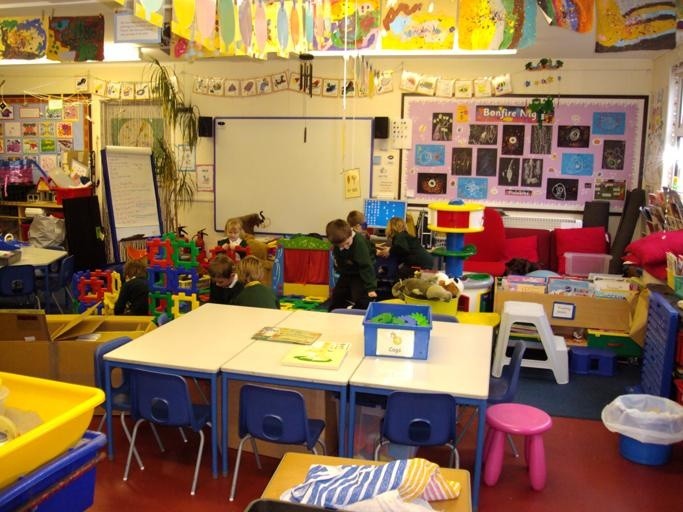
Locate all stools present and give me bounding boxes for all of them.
[492,300,571,385]
[482,404,552,490]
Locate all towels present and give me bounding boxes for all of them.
[291,458,462,512]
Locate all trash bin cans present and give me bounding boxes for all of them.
[614,393,683,466]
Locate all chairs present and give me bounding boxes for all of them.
[0,264,39,309]
[230,385,327,505]
[455,341,527,459]
[49,256,83,313]
[94,336,166,470]
[373,393,463,469]
[120,368,219,494]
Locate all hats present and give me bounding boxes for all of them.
[239,211,265,236]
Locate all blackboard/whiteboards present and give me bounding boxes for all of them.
[213,116,374,238]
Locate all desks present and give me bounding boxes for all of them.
[103,303,292,478]
[0,246,67,313]
[218,310,367,477]
[349,322,494,512]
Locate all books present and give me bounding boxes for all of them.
[282,343,352,371]
[501,272,630,300]
[252,326,321,345]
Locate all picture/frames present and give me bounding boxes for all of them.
[1,92,91,171]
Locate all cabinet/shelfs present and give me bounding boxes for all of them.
[0,200,66,244]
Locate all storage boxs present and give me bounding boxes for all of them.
[363,302,433,360]
[2,374,107,491]
[563,252,612,277]
[1,430,106,512]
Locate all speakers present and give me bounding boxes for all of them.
[197,117,213,138]
[375,116,390,140]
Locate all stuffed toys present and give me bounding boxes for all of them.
[413,269,465,298]
[392,277,452,302]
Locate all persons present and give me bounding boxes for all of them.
[326,218,378,312]
[383,217,435,284]
[114,260,148,316]
[217,219,247,252]
[234,254,280,310]
[348,211,377,264]
[206,254,245,304]
